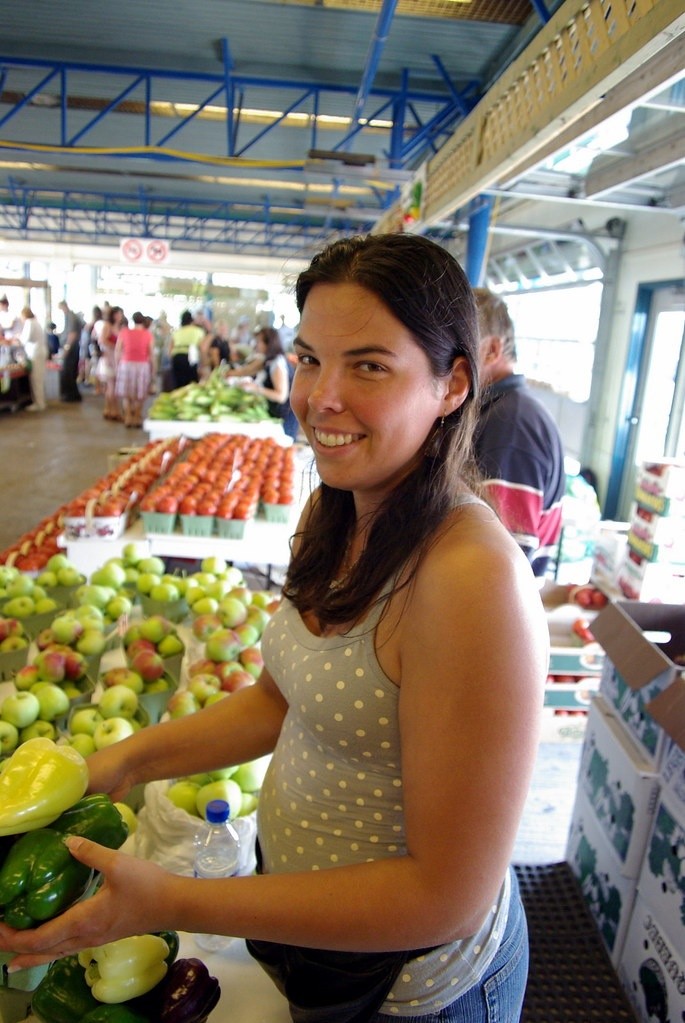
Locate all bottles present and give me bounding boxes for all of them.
[192,799,243,954]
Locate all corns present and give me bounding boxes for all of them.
[146,373,286,423]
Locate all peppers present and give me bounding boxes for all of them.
[0,737,222,1023]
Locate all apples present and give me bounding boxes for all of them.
[0,543,282,823]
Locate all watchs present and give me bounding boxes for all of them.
[64,345,71,350]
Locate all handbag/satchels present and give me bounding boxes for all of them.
[247,836,447,1023]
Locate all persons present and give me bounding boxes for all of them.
[209,323,236,371]
[0,232,550,1023]
[230,322,256,349]
[58,301,82,402]
[15,307,48,411]
[278,316,295,352]
[0,297,214,419]
[465,288,564,592]
[226,327,298,440]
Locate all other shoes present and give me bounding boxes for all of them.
[25,404,45,411]
[104,411,112,419]
[112,415,124,423]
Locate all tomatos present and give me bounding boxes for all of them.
[544,582,607,715]
[0,431,293,568]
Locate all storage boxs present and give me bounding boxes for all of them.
[534,461,685,1023]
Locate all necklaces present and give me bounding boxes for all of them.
[330,561,358,590]
